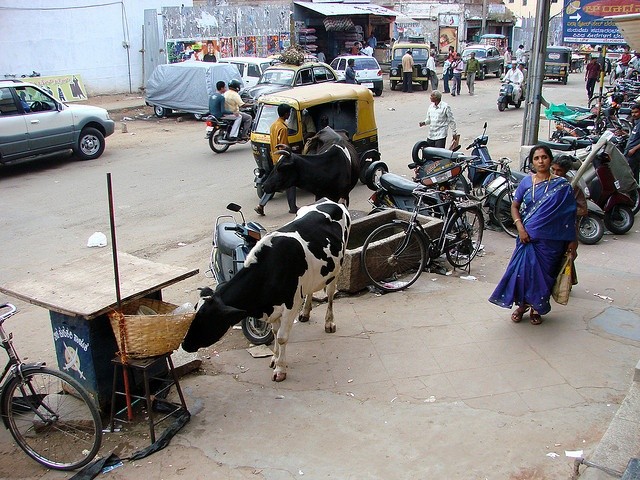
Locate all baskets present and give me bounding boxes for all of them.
[108,298,197,359]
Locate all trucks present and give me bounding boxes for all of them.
[389,37,430,91]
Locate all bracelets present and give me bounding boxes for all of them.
[513,219,522,225]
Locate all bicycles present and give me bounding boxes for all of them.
[0,301,102,470]
[537,80,640,150]
[607,62,622,85]
[360,186,484,292]
[425,154,526,239]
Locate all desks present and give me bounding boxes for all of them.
[0,249,199,430]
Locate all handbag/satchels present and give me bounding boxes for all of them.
[551,251,573,306]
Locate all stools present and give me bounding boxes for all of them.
[109,352,188,445]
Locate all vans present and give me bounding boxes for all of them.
[215,56,282,91]
[145,60,246,121]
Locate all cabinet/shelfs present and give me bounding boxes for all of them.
[326,30,365,59]
[298,31,318,53]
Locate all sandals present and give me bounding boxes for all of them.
[530,308,541,324]
[511,308,529,322]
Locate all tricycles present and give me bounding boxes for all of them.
[544,45,572,85]
[250,82,381,200]
[570,56,586,73]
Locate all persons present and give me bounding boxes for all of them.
[203,41,217,62]
[445,46,457,60]
[16,96,32,112]
[361,42,373,56]
[401,51,415,93]
[318,50,327,63]
[585,57,600,100]
[502,63,524,104]
[510,146,576,324]
[451,53,463,96]
[519,64,528,99]
[223,79,252,139]
[516,45,525,60]
[209,81,242,139]
[624,105,640,180]
[255,104,301,215]
[346,59,356,83]
[619,52,631,72]
[41,87,54,97]
[464,52,480,96]
[73,75,86,99]
[419,90,457,147]
[426,53,438,90]
[442,55,450,93]
[628,53,640,70]
[605,59,611,75]
[366,33,377,48]
[352,42,360,55]
[57,84,66,100]
[504,47,511,74]
[551,154,587,241]
[390,37,396,58]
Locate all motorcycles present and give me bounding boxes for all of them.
[496,77,525,111]
[365,145,481,236]
[585,139,634,235]
[408,121,498,199]
[482,138,604,245]
[518,130,640,218]
[204,100,258,153]
[204,203,273,346]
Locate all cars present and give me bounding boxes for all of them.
[0,82,115,168]
[246,62,339,101]
[460,45,504,80]
[329,56,384,96]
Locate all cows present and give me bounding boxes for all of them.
[261,125,361,209]
[181,196,352,383]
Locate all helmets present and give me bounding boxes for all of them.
[228,79,242,91]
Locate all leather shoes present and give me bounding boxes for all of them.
[254,205,265,216]
[288,207,299,213]
